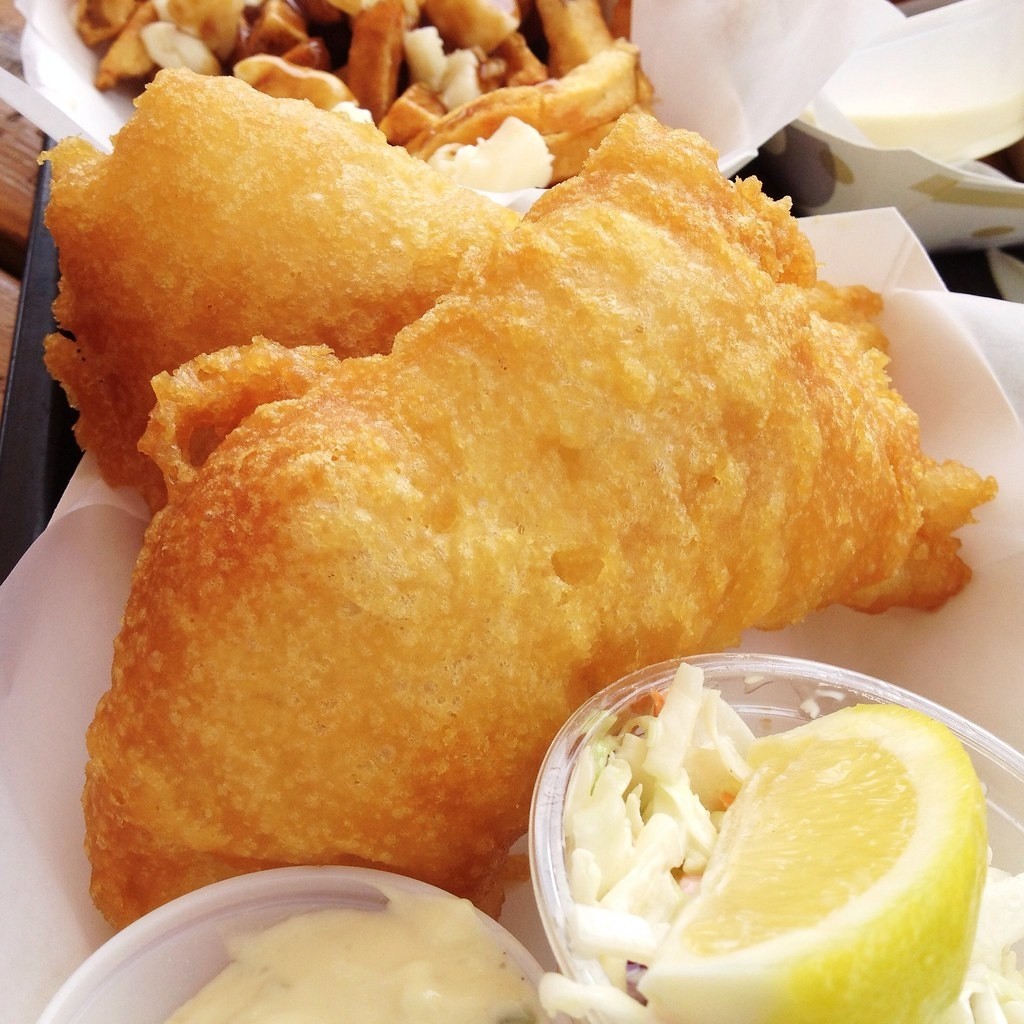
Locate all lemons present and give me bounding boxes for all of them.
[638,699,988,1024]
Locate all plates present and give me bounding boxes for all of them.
[48,207,1022,756]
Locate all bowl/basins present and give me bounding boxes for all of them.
[37,863,571,1024]
[20,2,869,215]
[528,654,1024,1022]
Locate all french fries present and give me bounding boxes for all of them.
[66,0,658,181]
